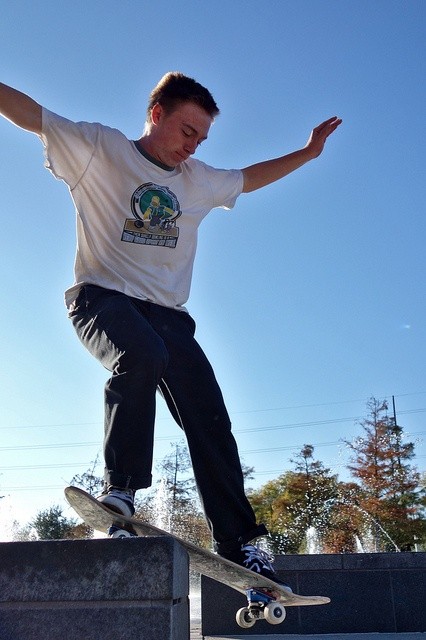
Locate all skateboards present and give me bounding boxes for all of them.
[64,486,331,628]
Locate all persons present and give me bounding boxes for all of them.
[0,72,342,594]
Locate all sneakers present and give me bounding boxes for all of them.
[96,483,134,517]
[218,544,291,592]
